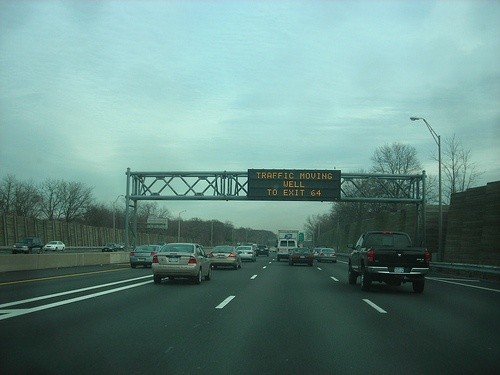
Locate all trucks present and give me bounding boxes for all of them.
[275,229,299,262]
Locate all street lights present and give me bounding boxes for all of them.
[409,114,444,261]
[232,230,237,241]
[210,219,218,247]
[310,230,315,248]
[113,194,124,243]
[316,221,320,237]
[246,229,252,242]
[177,209,187,243]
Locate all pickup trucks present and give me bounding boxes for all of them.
[347,230,432,294]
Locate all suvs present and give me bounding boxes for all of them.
[10,236,44,255]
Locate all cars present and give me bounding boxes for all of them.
[316,247,338,263]
[255,245,269,257]
[312,247,321,257]
[101,242,118,253]
[118,243,126,251]
[235,245,256,262]
[43,240,66,252]
[129,245,164,269]
[289,247,314,266]
[151,242,212,285]
[207,245,243,270]
[269,247,276,253]
